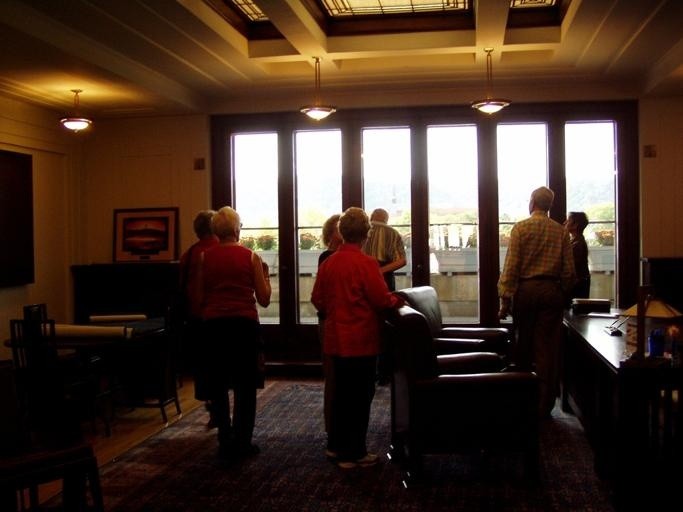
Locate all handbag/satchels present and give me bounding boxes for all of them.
[162,286,192,330]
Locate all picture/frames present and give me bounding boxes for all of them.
[113,207,180,263]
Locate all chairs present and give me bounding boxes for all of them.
[1,304,182,512]
[394,286,510,355]
[393,305,540,490]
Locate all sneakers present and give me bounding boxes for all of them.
[324,450,380,469]
[218,433,259,456]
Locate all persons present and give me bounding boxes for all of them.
[309,205,403,469]
[561,211,590,299]
[358,208,407,292]
[176,210,232,431]
[492,187,575,419]
[316,213,341,350]
[192,205,271,461]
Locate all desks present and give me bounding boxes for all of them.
[560,307,682,480]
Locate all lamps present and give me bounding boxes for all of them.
[471,47,511,115]
[300,56,338,122]
[59,89,93,134]
[620,259,682,369]
